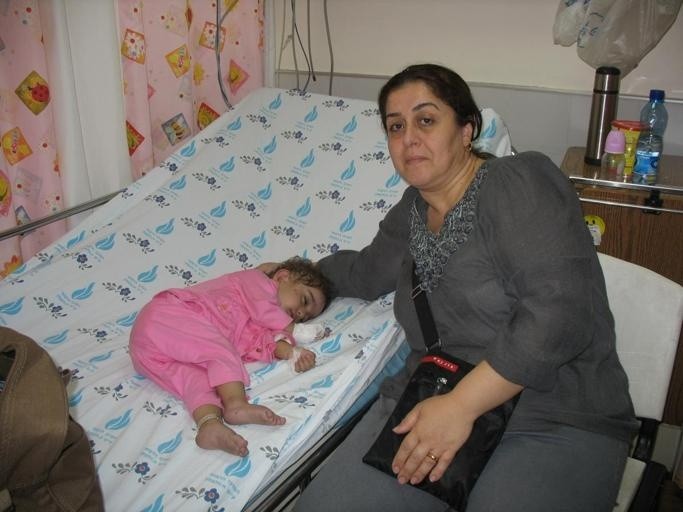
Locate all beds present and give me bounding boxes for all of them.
[0,87,513,512]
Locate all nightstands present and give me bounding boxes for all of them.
[559,146,683,429]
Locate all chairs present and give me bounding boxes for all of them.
[593,252,683,512]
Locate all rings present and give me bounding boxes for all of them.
[427,451,440,463]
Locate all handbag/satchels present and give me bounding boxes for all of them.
[362,345,522,511]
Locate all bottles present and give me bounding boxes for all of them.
[585,67,669,189]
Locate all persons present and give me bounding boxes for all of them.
[254,64,639,511]
[127,255,339,458]
